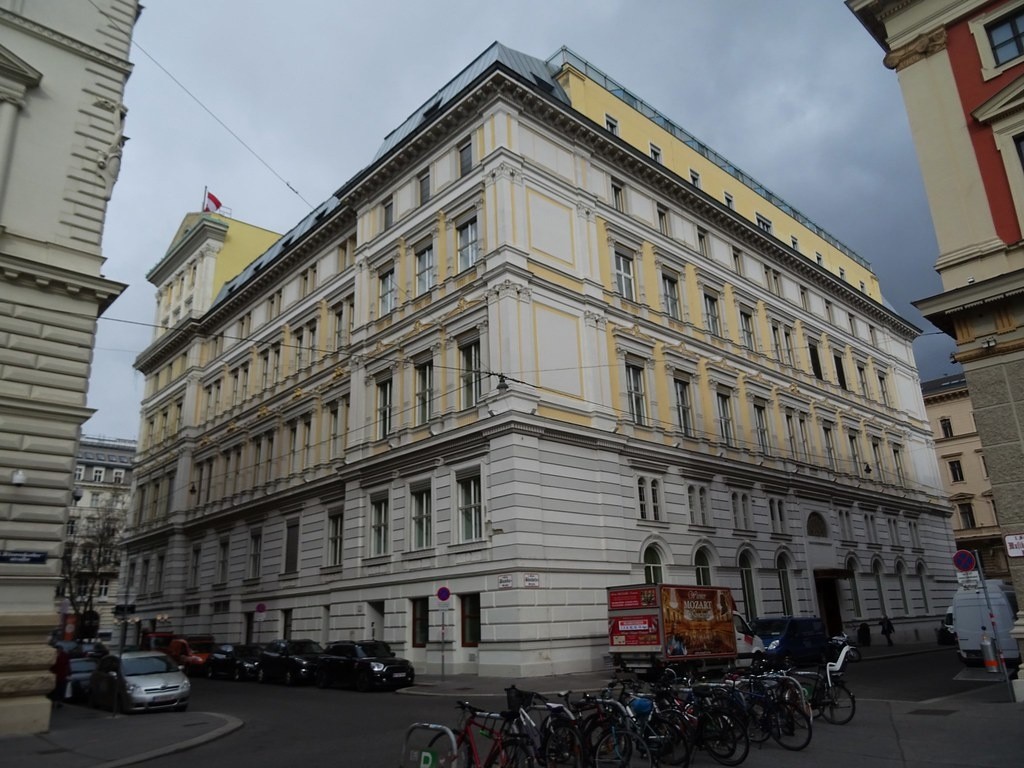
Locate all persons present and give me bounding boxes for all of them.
[878,613,896,647]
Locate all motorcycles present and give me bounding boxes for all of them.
[830,631,861,663]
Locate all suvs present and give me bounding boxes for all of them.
[139,632,216,676]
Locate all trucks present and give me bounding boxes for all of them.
[605,582,768,676]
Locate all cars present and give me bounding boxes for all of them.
[49,640,112,703]
[315,638,416,693]
[256,638,324,684]
[87,650,191,715]
[202,642,259,681]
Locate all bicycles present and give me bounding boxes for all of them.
[426,664,856,768]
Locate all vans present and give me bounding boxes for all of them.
[951,578,1022,666]
[747,615,828,673]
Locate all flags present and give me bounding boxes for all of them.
[204,192,222,212]
